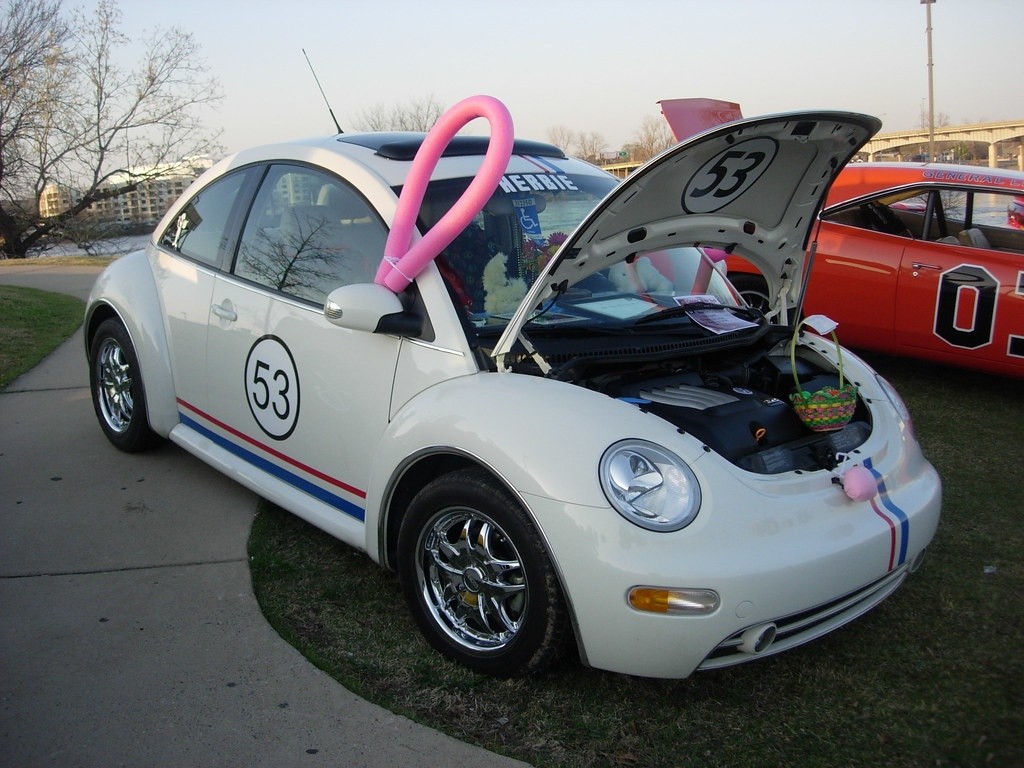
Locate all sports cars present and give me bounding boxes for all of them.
[82,109,943,683]
[656,96,1024,379]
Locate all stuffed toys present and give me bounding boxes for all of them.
[608,256,674,298]
[483,252,529,314]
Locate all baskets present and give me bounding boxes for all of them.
[789,317,859,433]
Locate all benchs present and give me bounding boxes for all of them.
[822,207,872,231]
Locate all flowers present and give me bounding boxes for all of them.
[520,231,570,278]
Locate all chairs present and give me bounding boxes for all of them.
[258,182,519,316]
[935,236,960,245]
[958,228,992,249]
